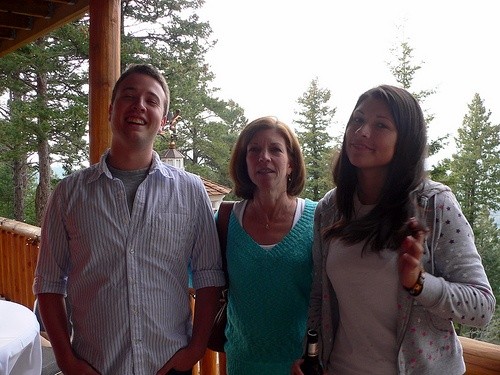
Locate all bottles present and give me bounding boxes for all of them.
[300,329,323,375]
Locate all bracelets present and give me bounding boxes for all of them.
[404,270,425,297]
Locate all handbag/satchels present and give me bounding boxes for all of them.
[205,289,227,352]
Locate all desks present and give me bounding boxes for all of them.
[0,299,43,375]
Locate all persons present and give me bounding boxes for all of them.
[291,84,498,375]
[212,117,319,375]
[35,63,227,375]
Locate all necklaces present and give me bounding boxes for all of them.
[255,196,287,231]
